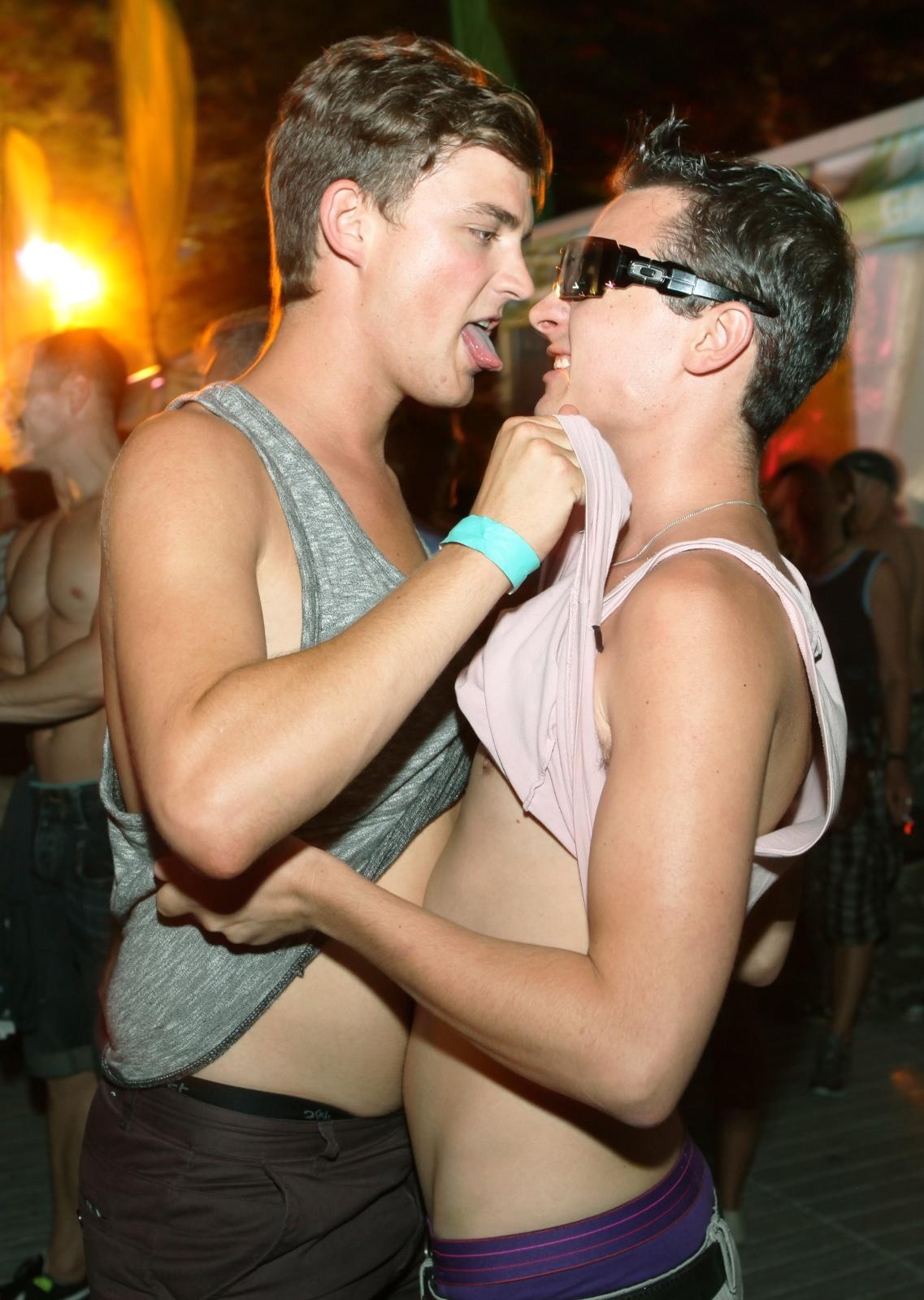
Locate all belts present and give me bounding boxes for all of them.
[602,1242,725,1300]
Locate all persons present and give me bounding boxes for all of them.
[152,109,848,1300]
[1,319,922,1300]
[77,32,628,1300]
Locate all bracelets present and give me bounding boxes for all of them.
[439,512,539,595]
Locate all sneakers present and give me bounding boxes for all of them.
[0,1254,90,1300]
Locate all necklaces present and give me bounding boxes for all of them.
[612,500,768,567]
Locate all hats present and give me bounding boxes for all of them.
[833,451,896,489]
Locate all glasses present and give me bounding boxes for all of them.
[555,235,780,320]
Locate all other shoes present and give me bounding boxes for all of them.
[814,1038,851,1100]
[722,1209,746,1244]
